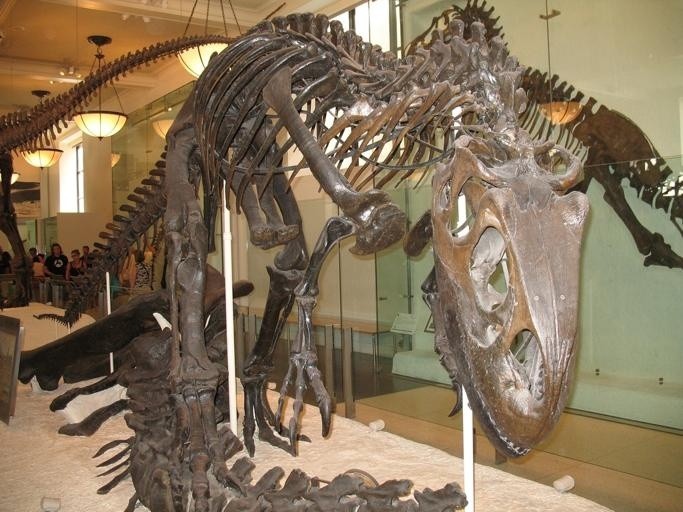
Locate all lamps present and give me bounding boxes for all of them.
[178,0,243,80]
[21,34,129,171]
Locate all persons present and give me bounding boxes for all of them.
[128,250,152,302]
[28,244,99,302]
[0,246,12,276]
